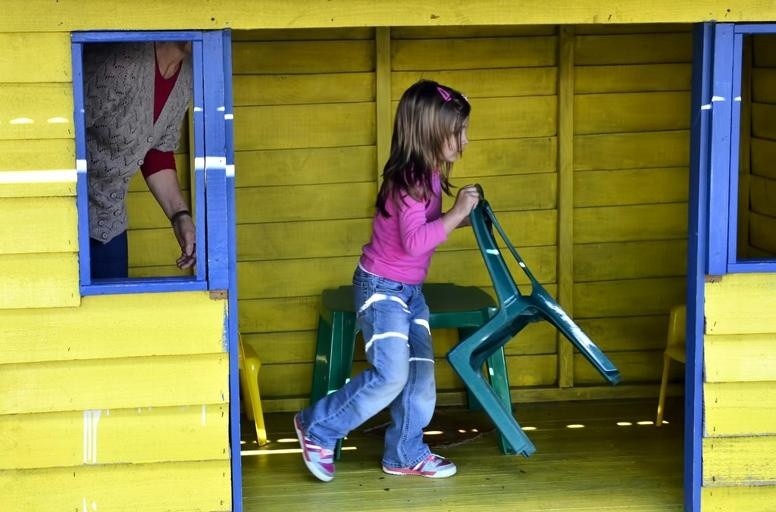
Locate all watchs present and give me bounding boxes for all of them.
[169,209,192,226]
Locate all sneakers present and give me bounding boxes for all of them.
[292,412,337,484]
[381,451,459,479]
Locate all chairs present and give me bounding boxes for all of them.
[238,329,272,447]
[446,183,623,459]
[656,303,685,427]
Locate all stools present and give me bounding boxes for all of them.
[310,282,518,455]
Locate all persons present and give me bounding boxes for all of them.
[82,40,198,278]
[293,79,486,484]
